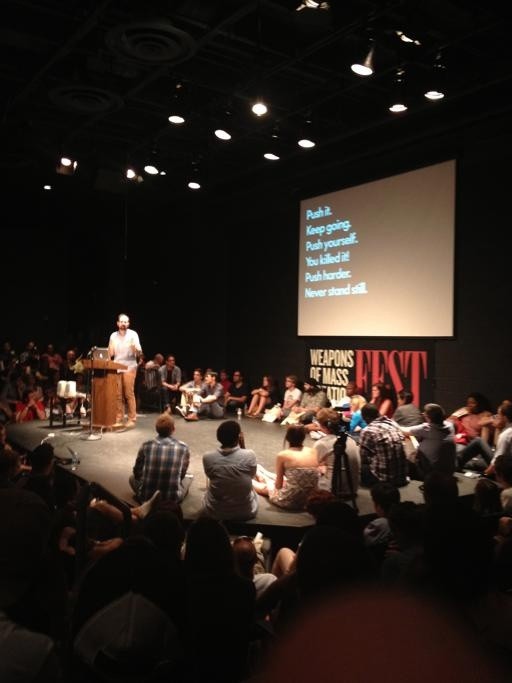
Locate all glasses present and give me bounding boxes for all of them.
[169,359,176,362]
[233,374,240,377]
[286,380,294,383]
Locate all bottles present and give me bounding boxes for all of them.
[237,408,242,416]
[71,451,79,471]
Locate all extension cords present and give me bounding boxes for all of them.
[48,432,54,438]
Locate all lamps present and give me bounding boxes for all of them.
[387,66,408,112]
[143,138,160,176]
[349,47,376,77]
[295,110,316,149]
[424,51,446,102]
[262,123,281,161]
[187,155,203,190]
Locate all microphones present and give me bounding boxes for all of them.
[87,345,97,358]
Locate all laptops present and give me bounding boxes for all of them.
[91,347,111,361]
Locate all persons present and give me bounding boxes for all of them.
[203,420,258,523]
[19,340,39,366]
[245,376,285,419]
[362,481,401,566]
[54,473,160,525]
[175,369,208,415]
[41,343,63,371]
[108,314,143,429]
[493,455,512,511]
[144,353,164,390]
[167,518,257,607]
[59,349,82,381]
[80,510,186,620]
[183,371,225,420]
[281,378,329,425]
[28,442,72,501]
[456,403,512,482]
[402,404,458,481]
[219,371,232,396]
[357,404,408,489]
[16,389,47,424]
[339,381,368,432]
[442,395,490,442]
[251,424,320,511]
[393,388,424,442]
[225,371,247,416]
[129,415,194,507]
[477,400,512,445]
[158,355,182,415]
[258,522,360,626]
[7,373,26,398]
[313,407,361,498]
[1,341,16,367]
[232,537,278,598]
[369,383,393,419]
[0,427,124,577]
[268,375,302,422]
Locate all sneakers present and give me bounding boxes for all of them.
[249,414,258,419]
[175,405,187,416]
[138,489,160,518]
[243,409,251,415]
[184,414,199,421]
[124,420,137,427]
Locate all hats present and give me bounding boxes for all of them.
[420,402,444,423]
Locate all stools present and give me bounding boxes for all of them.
[49,393,86,426]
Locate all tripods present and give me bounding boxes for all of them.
[321,416,375,512]
